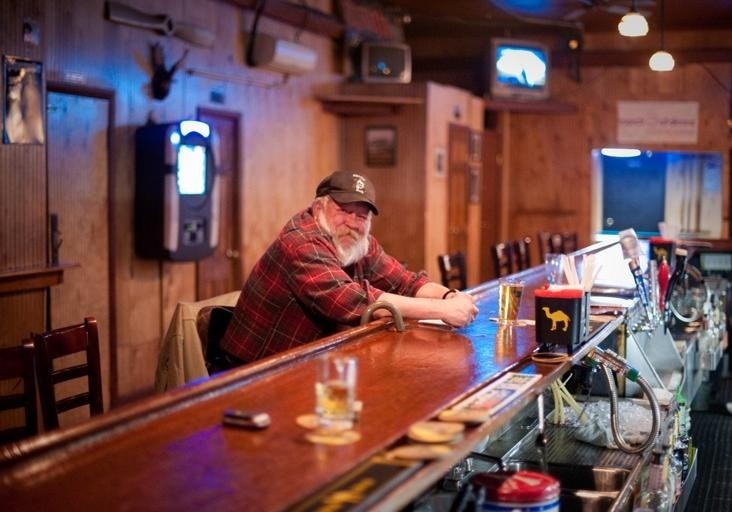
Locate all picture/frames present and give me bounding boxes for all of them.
[364,125,398,166]
[3,54,47,144]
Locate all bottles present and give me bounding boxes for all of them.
[701,293,729,370]
[637,401,696,511]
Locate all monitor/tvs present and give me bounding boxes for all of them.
[488,37,550,101]
[360,39,413,84]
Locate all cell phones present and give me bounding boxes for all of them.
[224,408,270,429]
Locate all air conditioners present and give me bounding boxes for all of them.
[252,31,316,75]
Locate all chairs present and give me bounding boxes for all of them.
[1,337,37,444]
[438,252,466,291]
[488,232,578,277]
[31,317,103,433]
[155,288,242,394]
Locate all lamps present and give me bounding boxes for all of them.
[618,0,650,38]
[649,0,675,72]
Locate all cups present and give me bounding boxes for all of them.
[495,321,518,365]
[544,252,563,285]
[314,351,356,435]
[498,276,523,319]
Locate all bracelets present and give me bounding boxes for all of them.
[442,288,457,300]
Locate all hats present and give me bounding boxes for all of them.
[315,169,379,216]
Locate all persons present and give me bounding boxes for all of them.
[208,169,480,378]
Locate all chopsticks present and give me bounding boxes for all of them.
[561,254,603,292]
